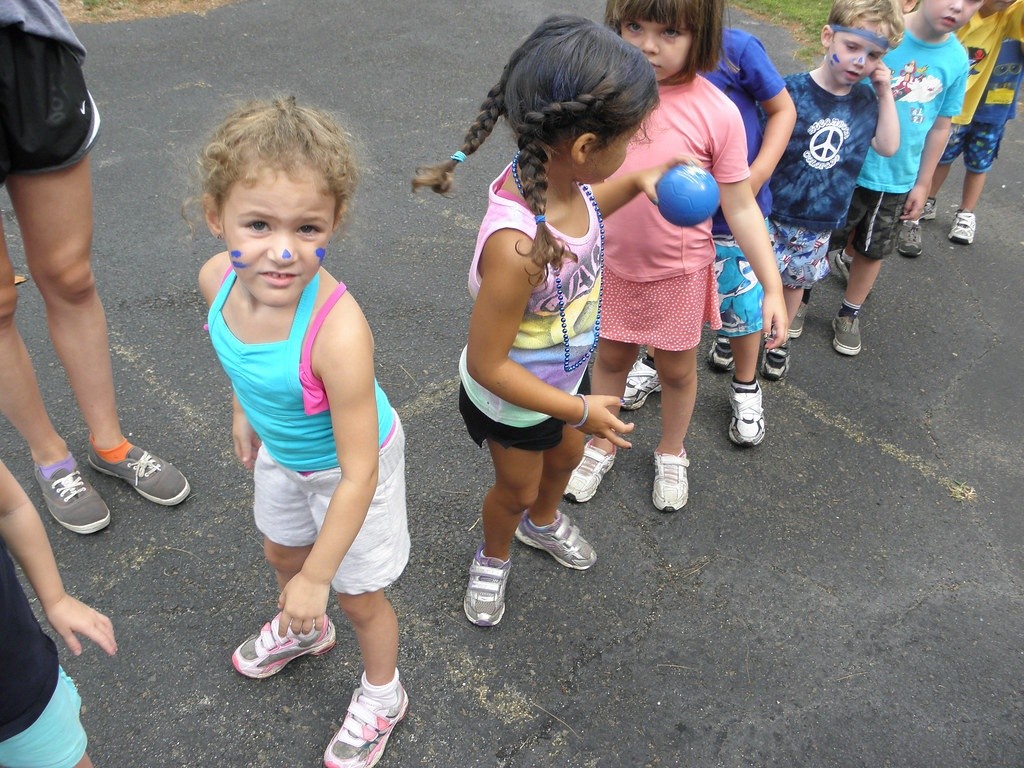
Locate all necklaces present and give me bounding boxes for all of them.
[513,148,605,371]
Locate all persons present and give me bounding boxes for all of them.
[197,92,411,768]
[621,27,797,444]
[708,0,902,382]
[919,36,1024,244]
[835,0,1024,297]
[0,457,119,768]
[0,0,190,536]
[788,0,986,357]
[562,0,790,513]
[413,14,704,628]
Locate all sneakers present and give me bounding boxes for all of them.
[948,211,976,244]
[324,681,409,768]
[835,249,853,282]
[760,335,791,380]
[232,611,335,679]
[832,311,861,355]
[787,300,808,338]
[514,509,597,570]
[562,438,616,503]
[912,200,937,225]
[621,358,662,410]
[34,463,110,534]
[652,446,690,512]
[464,544,511,626]
[88,443,191,506]
[896,221,923,256]
[728,376,765,447]
[709,335,736,371]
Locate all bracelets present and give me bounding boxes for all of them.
[566,394,589,428]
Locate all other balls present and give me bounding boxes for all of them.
[656,163,721,227]
